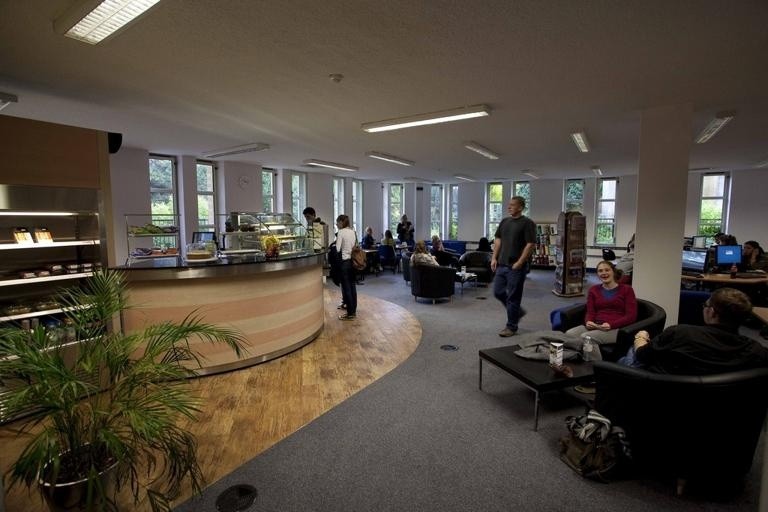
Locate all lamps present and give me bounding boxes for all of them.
[694,112,734,145]
[53,0,162,49]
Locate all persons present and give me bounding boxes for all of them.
[336,283,347,309]
[410,238,440,267]
[431,235,446,252]
[475,237,493,252]
[301,206,326,250]
[361,224,376,250]
[335,214,358,322]
[714,232,725,244]
[490,195,538,337]
[381,230,397,251]
[618,286,765,378]
[730,240,767,273]
[563,259,639,394]
[396,213,416,245]
[718,235,737,246]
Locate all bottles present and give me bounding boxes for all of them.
[583,336,593,361]
[730,262,738,279]
[17,312,103,352]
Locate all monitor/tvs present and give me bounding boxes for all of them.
[682,250,710,273]
[193,232,215,243]
[716,245,742,274]
[693,236,706,249]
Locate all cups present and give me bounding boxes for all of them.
[461,266,467,273]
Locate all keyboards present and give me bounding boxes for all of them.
[682,270,701,277]
[734,272,761,278]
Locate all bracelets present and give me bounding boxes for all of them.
[632,336,649,343]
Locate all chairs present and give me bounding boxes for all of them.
[560,299,666,361]
[378,245,495,305]
[602,249,618,267]
[592,326,768,490]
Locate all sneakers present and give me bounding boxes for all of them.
[336,302,347,309]
[338,314,355,321]
[519,307,527,319]
[498,326,515,337]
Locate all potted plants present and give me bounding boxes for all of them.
[0,266,250,512]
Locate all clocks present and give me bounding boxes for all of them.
[239,175,251,190]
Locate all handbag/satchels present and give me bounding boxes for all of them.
[557,425,630,486]
[352,245,367,271]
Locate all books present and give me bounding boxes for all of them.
[532,215,588,296]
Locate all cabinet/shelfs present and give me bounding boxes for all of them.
[0,210,114,422]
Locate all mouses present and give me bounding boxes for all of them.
[697,275,704,278]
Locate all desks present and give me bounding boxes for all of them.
[361,249,379,280]
[682,271,768,291]
